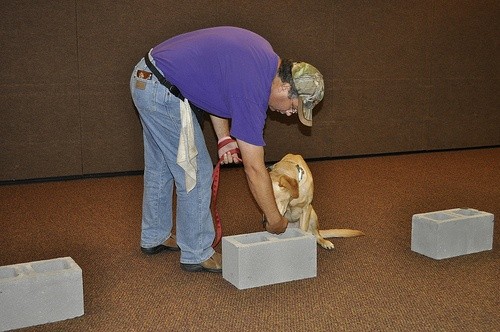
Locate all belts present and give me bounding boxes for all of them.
[145,51,184,102]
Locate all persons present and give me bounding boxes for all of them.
[130,27,324,272]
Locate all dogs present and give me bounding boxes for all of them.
[266,154,365,249]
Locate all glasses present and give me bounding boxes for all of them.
[292,103,297,114]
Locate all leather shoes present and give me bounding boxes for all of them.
[141,234,181,254]
[180,252,222,273]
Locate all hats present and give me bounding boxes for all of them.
[291,61,324,126]
